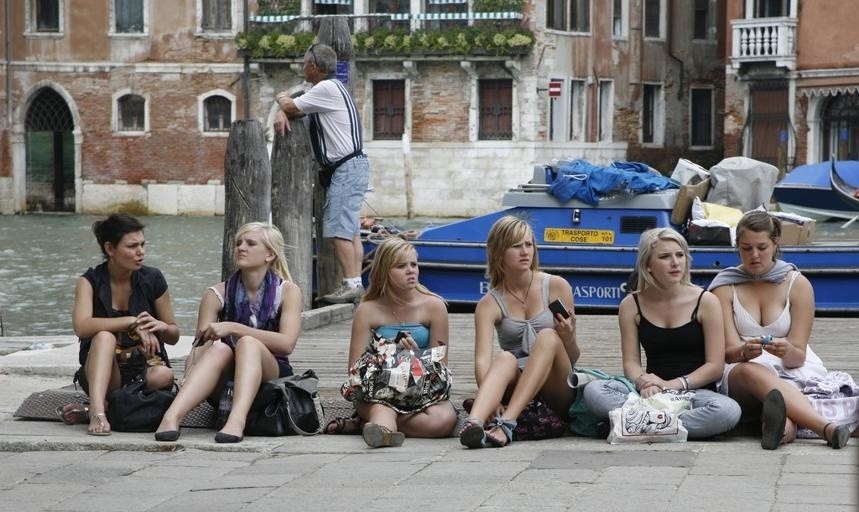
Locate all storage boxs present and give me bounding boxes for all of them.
[773,211,817,246]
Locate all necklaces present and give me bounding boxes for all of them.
[386,296,416,329]
[503,268,534,305]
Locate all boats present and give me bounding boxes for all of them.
[311,165,859,317]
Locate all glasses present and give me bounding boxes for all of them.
[309,44,319,67]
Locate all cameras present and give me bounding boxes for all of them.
[546,296,571,322]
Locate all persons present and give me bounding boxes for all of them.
[156,221,303,443]
[584,229,741,440]
[457,213,580,447]
[273,43,370,303]
[55,214,180,436]
[706,214,851,452]
[324,237,459,446]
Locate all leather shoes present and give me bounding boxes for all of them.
[214,431,243,443]
[155,425,181,441]
[823,421,853,450]
[760,389,787,449]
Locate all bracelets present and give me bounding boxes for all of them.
[677,375,690,390]
[277,95,285,103]
[127,318,142,333]
[741,341,747,361]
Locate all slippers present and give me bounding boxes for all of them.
[55,402,88,425]
[87,413,114,436]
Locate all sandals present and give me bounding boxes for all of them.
[460,415,488,449]
[326,416,360,434]
[363,422,405,447]
[486,415,518,447]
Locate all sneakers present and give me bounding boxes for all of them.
[314,281,367,300]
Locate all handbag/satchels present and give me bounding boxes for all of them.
[567,367,640,438]
[462,396,565,440]
[340,331,453,414]
[106,383,179,433]
[606,392,690,444]
[318,166,331,189]
[243,369,325,436]
[777,360,859,440]
[177,378,219,430]
